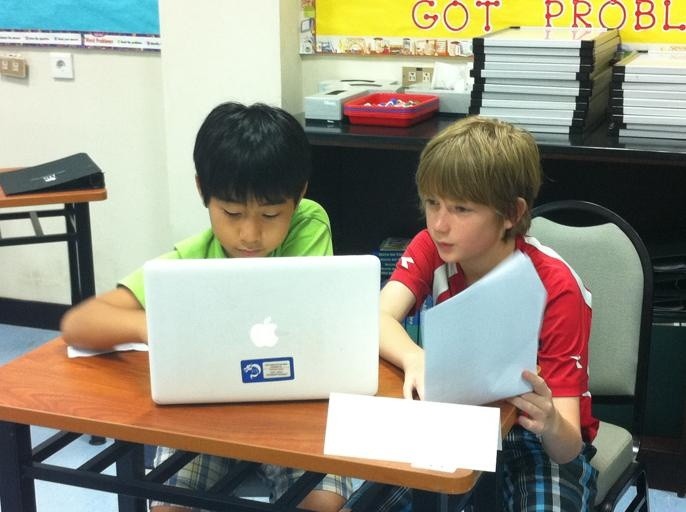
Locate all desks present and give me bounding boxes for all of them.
[0,327,543,512]
[0,163,110,445]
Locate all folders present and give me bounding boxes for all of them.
[1,152,105,196]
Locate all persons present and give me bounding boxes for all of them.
[338,115,599,511]
[58,101,354,512]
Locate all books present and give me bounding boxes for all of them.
[468,26,686,148]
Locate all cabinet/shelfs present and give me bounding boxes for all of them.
[293,110,686,501]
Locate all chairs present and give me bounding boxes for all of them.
[511,197,658,512]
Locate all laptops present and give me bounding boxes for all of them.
[143,254,381,405]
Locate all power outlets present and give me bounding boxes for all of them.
[402,66,434,88]
[0,57,28,80]
